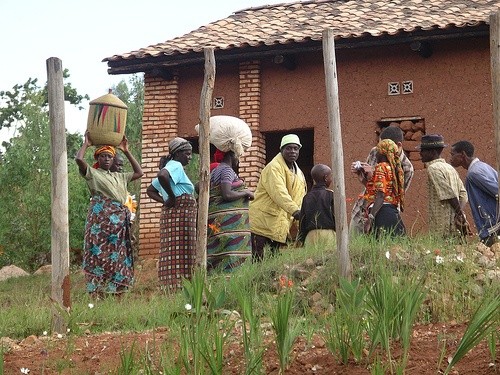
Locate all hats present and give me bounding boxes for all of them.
[280,134,302,150]
[415,134,448,149]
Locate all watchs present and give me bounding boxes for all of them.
[368,214,374,219]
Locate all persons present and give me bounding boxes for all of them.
[362,139,406,241]
[249,134,307,264]
[158,154,173,170]
[75,130,144,298]
[420,135,473,245]
[351,126,414,234]
[451,141,500,247]
[195,150,254,273]
[147,137,197,293]
[298,164,337,245]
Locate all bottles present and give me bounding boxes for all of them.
[130,193,136,225]
